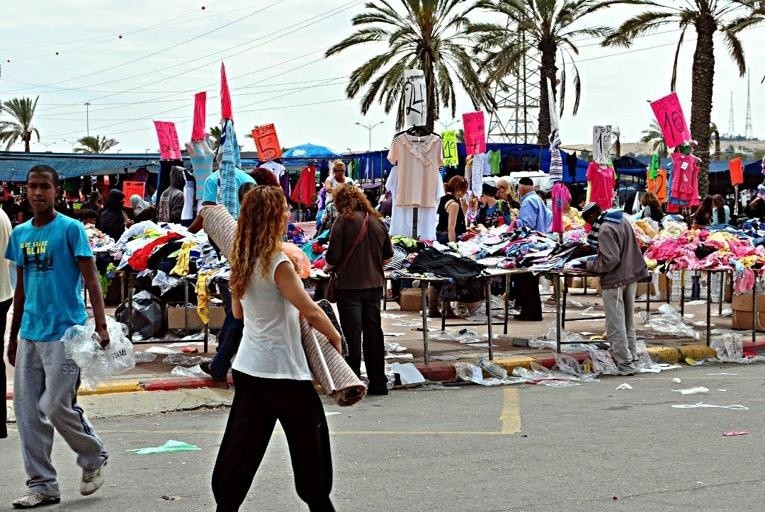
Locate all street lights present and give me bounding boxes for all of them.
[82,102,90,151]
[346,148,351,154]
[145,149,151,154]
[434,117,460,129]
[382,146,387,150]
[116,149,121,154]
[354,120,384,151]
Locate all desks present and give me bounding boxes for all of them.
[83,238,765,364]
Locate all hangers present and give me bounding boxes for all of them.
[405,123,431,136]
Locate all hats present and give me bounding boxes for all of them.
[482,183,499,196]
[169,166,187,177]
[582,202,596,216]
[519,177,533,185]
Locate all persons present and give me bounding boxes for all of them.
[323,160,354,211]
[0,164,111,508]
[322,184,393,397]
[640,191,765,230]
[208,182,257,389]
[81,166,187,240]
[210,184,341,512]
[427,175,579,321]
[573,202,650,366]
[188,147,258,236]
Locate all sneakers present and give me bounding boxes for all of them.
[367,376,388,395]
[80,458,113,495]
[468,303,482,313]
[612,358,640,376]
[200,360,227,381]
[514,312,543,321]
[0,427,7,439]
[13,491,60,508]
[426,309,460,319]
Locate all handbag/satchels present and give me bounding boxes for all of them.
[324,274,338,303]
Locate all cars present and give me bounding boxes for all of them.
[582,178,641,203]
